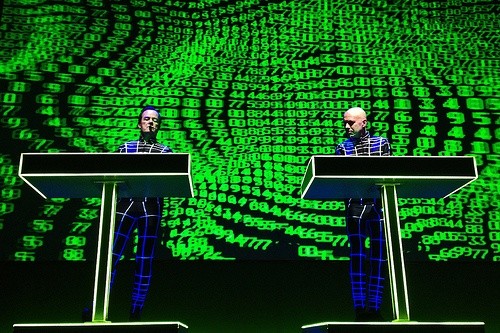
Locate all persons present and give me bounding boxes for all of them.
[334,107,393,322]
[81,107,164,321]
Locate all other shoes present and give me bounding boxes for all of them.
[356,310,385,321]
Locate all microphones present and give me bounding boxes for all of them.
[358,132,365,155]
